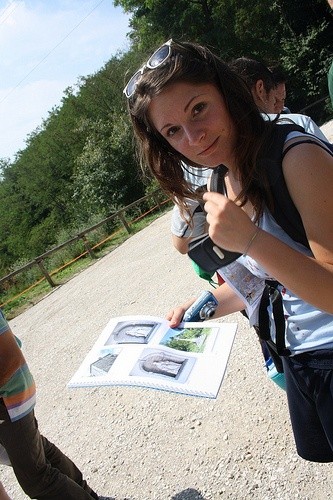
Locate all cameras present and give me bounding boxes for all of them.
[181,290,219,323]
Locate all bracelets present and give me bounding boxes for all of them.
[243,227,262,257]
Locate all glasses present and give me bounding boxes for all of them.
[120,38,203,99]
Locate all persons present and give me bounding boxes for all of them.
[127,40,333,500]
[0,310,99,500]
